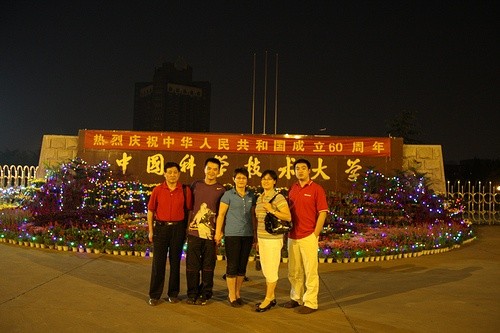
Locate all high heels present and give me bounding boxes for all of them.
[227,296,240,307]
[238,298,244,305]
[256,299,276,307]
[255,301,272,312]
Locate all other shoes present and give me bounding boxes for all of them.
[196,295,212,305]
[282,300,298,308]
[169,297,177,303]
[149,298,158,305]
[298,306,317,314]
[186,297,196,304]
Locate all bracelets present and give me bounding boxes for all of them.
[273,210,276,214]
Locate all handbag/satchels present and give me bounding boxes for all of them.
[265,194,292,234]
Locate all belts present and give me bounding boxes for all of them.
[157,221,183,226]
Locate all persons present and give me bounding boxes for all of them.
[283,159,328,314]
[185,157,227,304]
[147,161,192,305]
[255,170,292,312]
[214,166,255,307]
